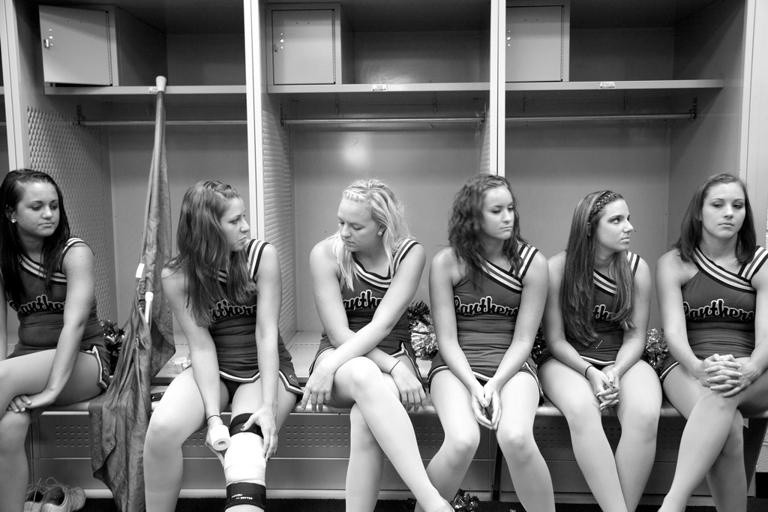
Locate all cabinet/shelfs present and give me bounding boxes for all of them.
[0,1,757,500]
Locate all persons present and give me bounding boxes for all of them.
[537,187,664,512]
[1,168,119,512]
[651,173,767,511]
[412,172,555,512]
[140,176,305,512]
[300,176,456,512]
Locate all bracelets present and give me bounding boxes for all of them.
[582,362,595,380]
[202,414,222,424]
[387,358,402,377]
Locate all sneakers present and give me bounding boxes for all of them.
[24,486,43,512]
[42,485,86,512]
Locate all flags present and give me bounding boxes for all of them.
[86,86,174,512]
